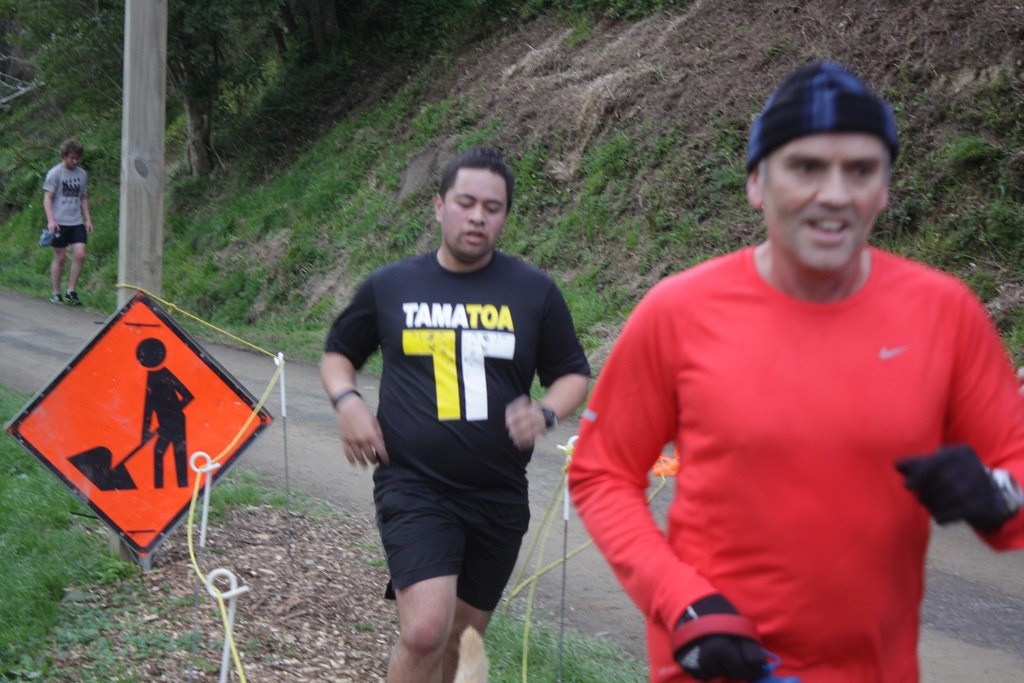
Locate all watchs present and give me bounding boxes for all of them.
[543,408,558,429]
[990,467,1024,522]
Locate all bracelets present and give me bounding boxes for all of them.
[672,613,760,658]
[334,389,361,408]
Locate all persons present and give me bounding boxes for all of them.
[318,145,591,683]
[569,60,1024,683]
[41,138,93,306]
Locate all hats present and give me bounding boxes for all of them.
[745,59,898,179]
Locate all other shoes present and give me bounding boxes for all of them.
[50,293,64,305]
[64,291,81,306]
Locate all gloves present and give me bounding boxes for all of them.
[669,592,771,683]
[895,443,1020,538]
